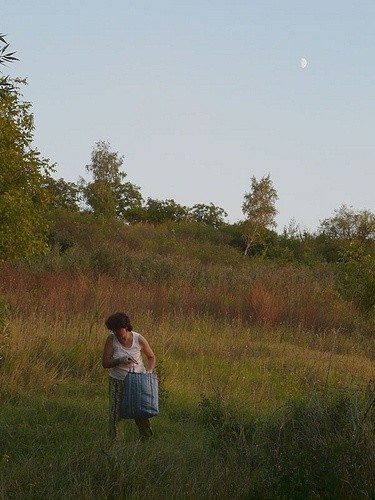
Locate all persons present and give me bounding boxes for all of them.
[101,312,157,437]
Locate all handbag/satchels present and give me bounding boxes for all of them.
[120,363,158,419]
